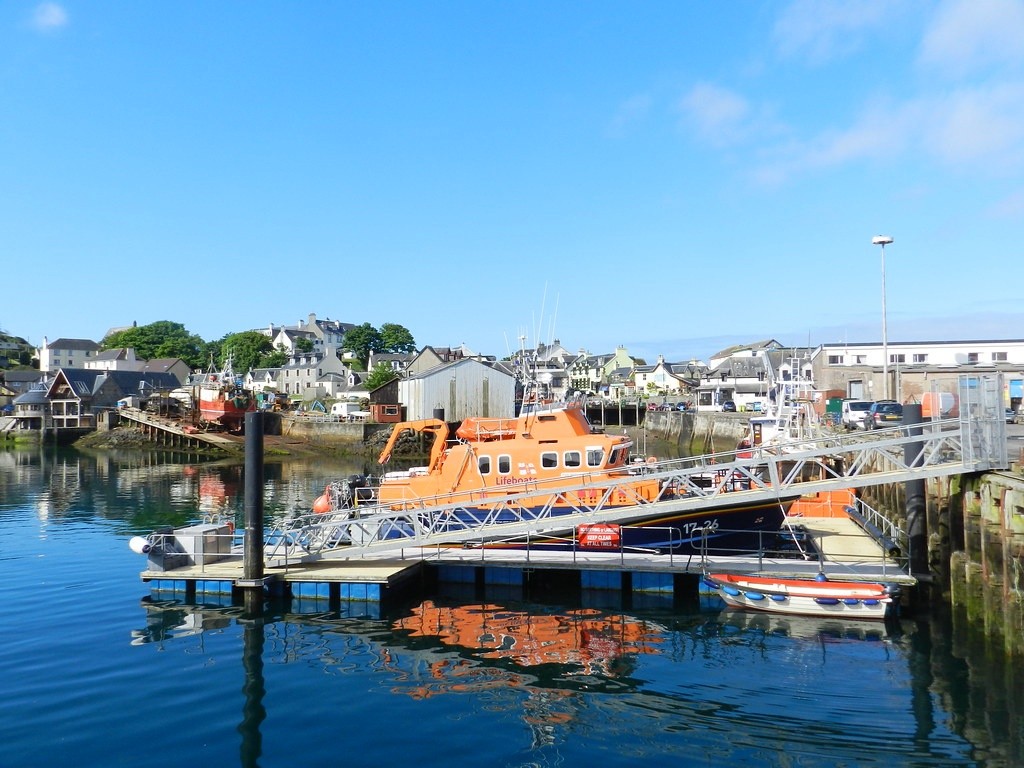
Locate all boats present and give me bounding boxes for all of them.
[714,603,902,643]
[184,406,857,558]
[186,347,259,432]
[702,569,901,619]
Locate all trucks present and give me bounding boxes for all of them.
[331,402,360,419]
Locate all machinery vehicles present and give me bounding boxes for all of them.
[303,400,327,418]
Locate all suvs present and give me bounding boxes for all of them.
[863,401,906,430]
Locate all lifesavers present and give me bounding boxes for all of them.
[648,457,657,462]
[225,522,233,535]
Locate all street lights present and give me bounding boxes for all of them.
[873,235,892,400]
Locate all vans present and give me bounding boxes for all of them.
[921,391,973,422]
[843,399,873,429]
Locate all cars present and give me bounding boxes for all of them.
[989,408,1019,425]
[676,402,688,411]
[659,403,675,412]
[722,402,736,413]
[649,402,658,411]
